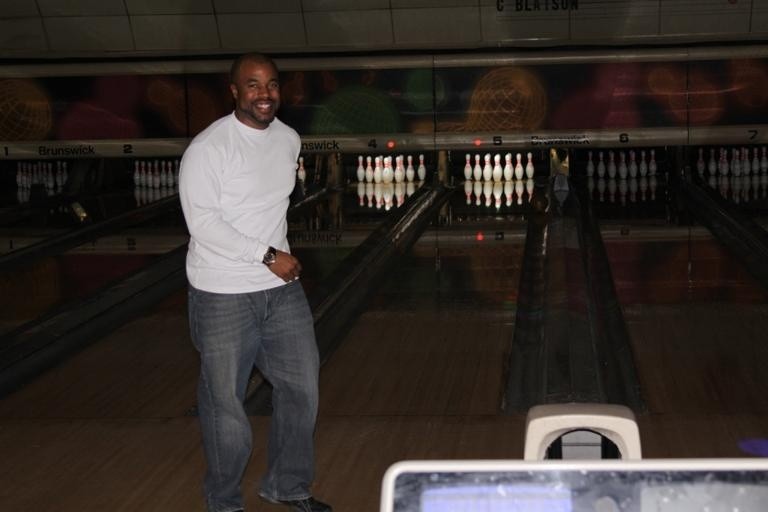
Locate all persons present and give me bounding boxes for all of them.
[176,49,337,511]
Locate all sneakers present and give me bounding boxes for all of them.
[256,485,333,512]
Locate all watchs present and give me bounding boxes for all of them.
[261,246,277,265]
